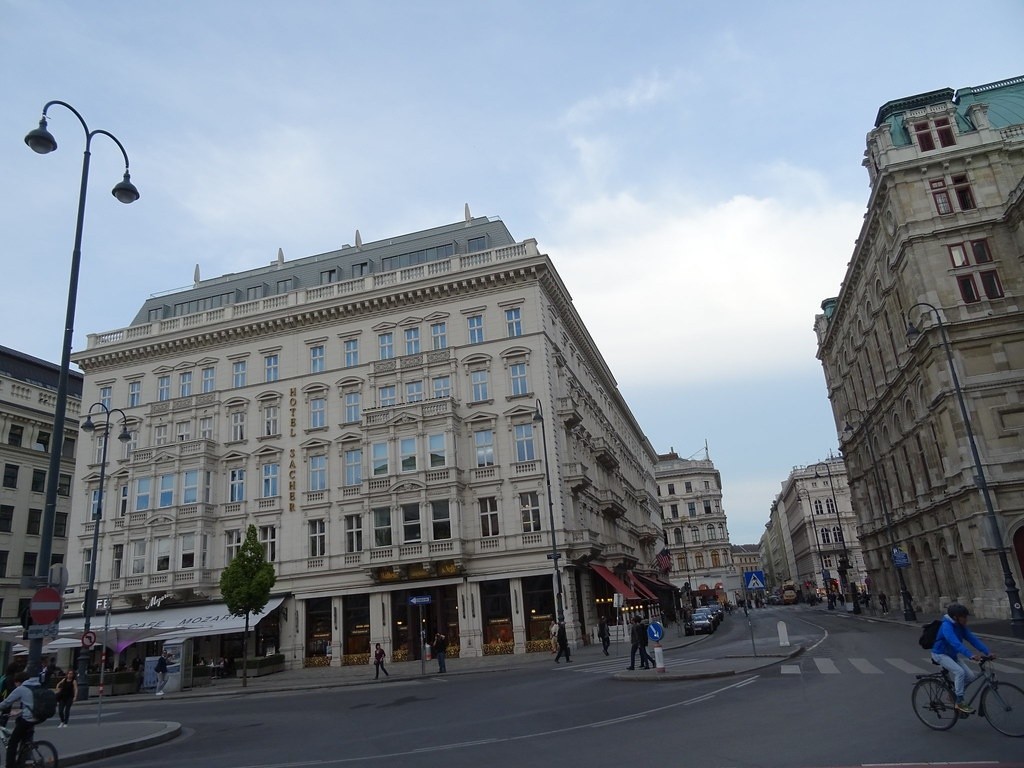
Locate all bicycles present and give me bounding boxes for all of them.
[0,714,59,768]
[911,655,1024,738]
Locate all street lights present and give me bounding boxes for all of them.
[681,518,693,610]
[797,488,835,610]
[814,462,861,614]
[844,409,917,621]
[904,302,1024,640]
[532,398,571,657]
[25,100,140,676]
[75,403,132,701]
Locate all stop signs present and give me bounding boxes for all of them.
[30,588,62,625]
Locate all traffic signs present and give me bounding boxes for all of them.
[409,596,431,605]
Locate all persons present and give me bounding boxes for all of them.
[549,621,561,653]
[931,604,995,713]
[598,616,611,655]
[375,643,389,679]
[806,589,914,616]
[0,650,228,767]
[433,633,448,673]
[627,616,656,670]
[554,622,573,664]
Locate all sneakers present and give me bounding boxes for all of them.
[954,701,976,715]
[946,694,967,705]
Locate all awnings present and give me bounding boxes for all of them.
[49,597,287,650]
[624,569,658,601]
[589,563,642,599]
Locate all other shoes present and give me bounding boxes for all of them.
[551,651,556,654]
[155,691,164,696]
[58,722,64,728]
[643,667,650,669]
[373,678,378,680]
[626,667,634,670]
[639,666,644,668]
[388,675,390,678]
[63,723,68,728]
[553,659,560,664]
[653,663,656,667]
[438,671,446,673]
[602,650,609,656]
[566,661,574,663]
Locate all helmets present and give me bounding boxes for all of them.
[948,604,969,621]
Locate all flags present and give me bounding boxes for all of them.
[655,547,672,571]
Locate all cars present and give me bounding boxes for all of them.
[708,605,725,621]
[694,608,719,630]
[683,613,713,636]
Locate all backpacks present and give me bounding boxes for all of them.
[918,620,942,649]
[23,683,57,720]
